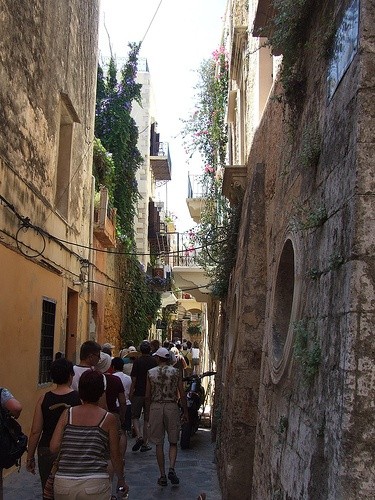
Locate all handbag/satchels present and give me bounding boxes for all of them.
[43,461,58,500]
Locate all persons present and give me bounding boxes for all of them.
[144,348,189,486]
[25,358,81,489]
[49,369,128,500]
[0,386,23,500]
[71,338,200,464]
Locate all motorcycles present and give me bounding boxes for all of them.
[180,371,217,449]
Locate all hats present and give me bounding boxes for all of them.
[47,358,76,377]
[101,342,115,351]
[152,347,170,358]
[128,346,138,353]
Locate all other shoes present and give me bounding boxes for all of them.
[132,436,144,451]
[157,476,168,486]
[140,444,153,452]
[167,468,180,485]
[116,482,129,500]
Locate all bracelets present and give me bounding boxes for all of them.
[118,477,125,481]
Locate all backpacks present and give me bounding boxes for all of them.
[0,386,29,470]
[181,351,189,366]
